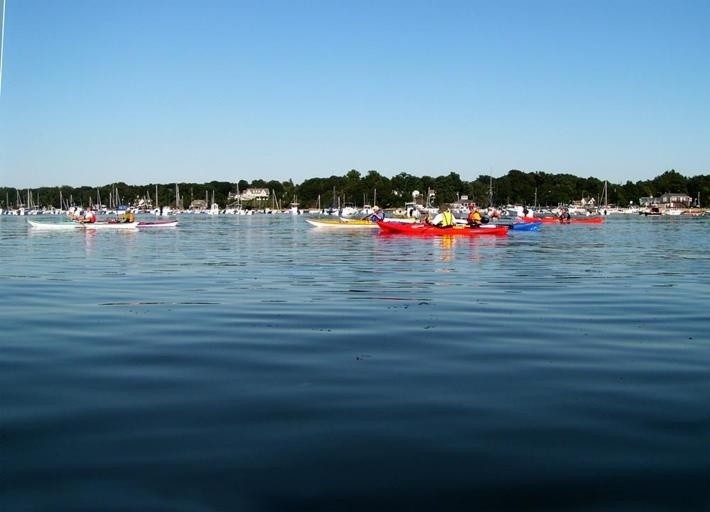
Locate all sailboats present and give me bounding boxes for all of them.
[0,186,300,215]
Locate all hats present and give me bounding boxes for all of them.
[467,202,477,208]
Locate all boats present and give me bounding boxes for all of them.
[23,217,181,231]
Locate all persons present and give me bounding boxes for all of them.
[67,203,663,229]
